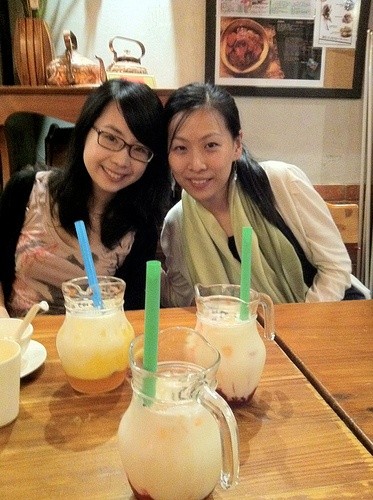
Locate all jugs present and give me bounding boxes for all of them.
[55,275,135,394]
[193,282,276,408]
[115,325,240,500]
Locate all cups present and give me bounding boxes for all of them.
[0,340,22,429]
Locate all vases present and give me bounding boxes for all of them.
[14,18,54,87]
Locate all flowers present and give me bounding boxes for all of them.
[22,0,47,20]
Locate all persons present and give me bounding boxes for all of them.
[0,78,171,319]
[160,80,370,308]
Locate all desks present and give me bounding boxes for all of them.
[0,299,373,500]
[0,87,184,188]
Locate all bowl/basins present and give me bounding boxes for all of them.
[0,318,35,356]
[219,18,271,74]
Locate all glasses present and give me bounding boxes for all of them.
[92,124,154,163]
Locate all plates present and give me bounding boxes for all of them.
[20,339,47,379]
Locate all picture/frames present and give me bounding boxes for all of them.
[205,0,370,99]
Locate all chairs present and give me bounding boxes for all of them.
[324,202,372,301]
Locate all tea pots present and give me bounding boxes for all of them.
[46,30,101,86]
[93,35,152,86]
